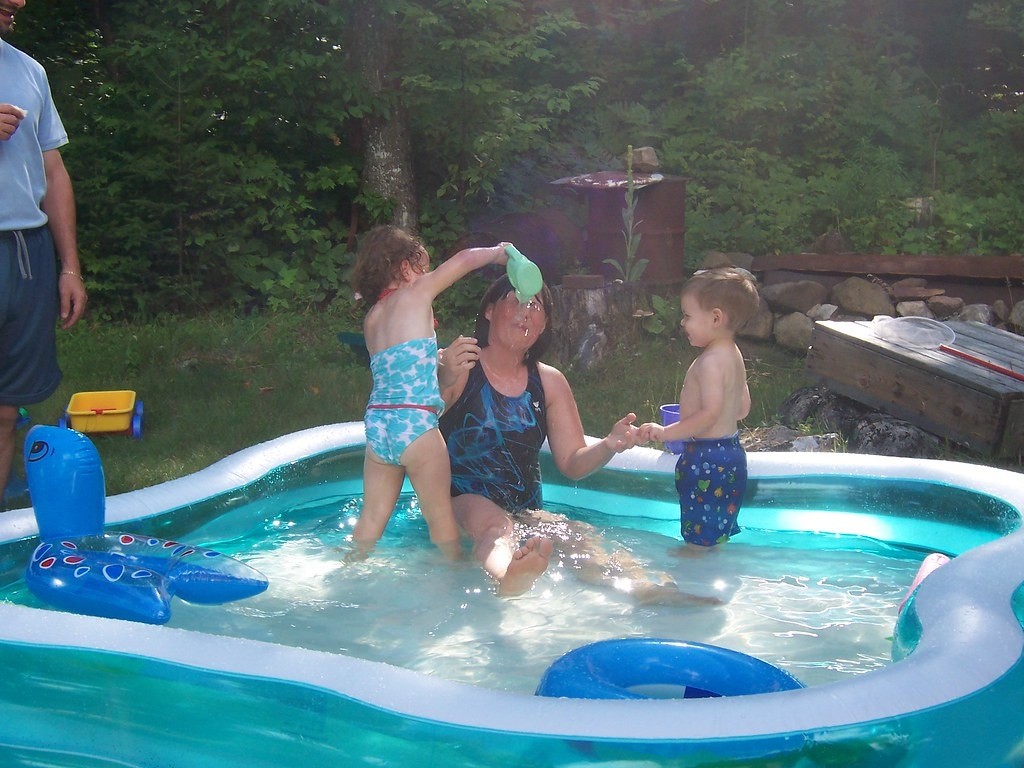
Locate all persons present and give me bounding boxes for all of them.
[438,270,640,595]
[637,268,756,549]
[345,225,517,547]
[0,0,88,499]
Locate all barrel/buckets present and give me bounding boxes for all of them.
[661,404,692,455]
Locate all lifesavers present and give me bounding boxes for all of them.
[532,633,815,699]
[22,423,270,626]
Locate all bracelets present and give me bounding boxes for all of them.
[61,271,83,280]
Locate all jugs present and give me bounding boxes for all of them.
[506,242,542,306]
